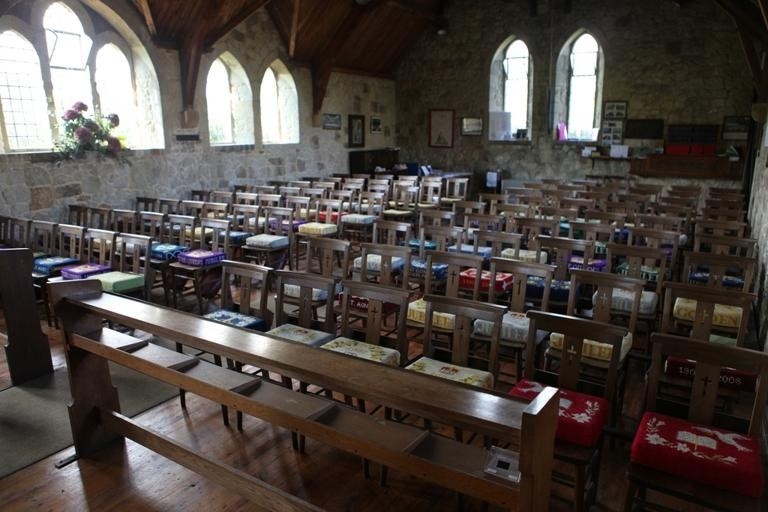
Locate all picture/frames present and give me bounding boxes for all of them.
[721,115,752,140]
[348,114,365,148]
[370,116,384,134]
[322,113,341,130]
[603,100,628,119]
[428,108,455,149]
[460,117,484,136]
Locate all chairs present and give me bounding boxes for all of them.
[100,232,155,330]
[146,197,174,225]
[390,224,461,299]
[507,309,628,512]
[169,217,231,314]
[591,243,669,378]
[541,266,648,415]
[47,228,120,330]
[124,193,144,220]
[513,235,605,315]
[176,199,200,245]
[642,280,755,412]
[668,249,756,342]
[470,256,559,384]
[7,217,29,250]
[298,280,415,453]
[333,242,413,319]
[423,167,756,248]
[233,172,420,248]
[380,292,510,511]
[67,203,85,251]
[406,249,484,360]
[622,331,768,511]
[89,204,109,263]
[115,210,167,258]
[174,258,275,430]
[30,224,88,303]
[273,238,351,324]
[457,231,524,307]
[236,268,337,452]
[139,214,197,305]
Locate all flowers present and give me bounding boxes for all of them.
[55,102,134,168]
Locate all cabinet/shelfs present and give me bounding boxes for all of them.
[349,148,398,173]
